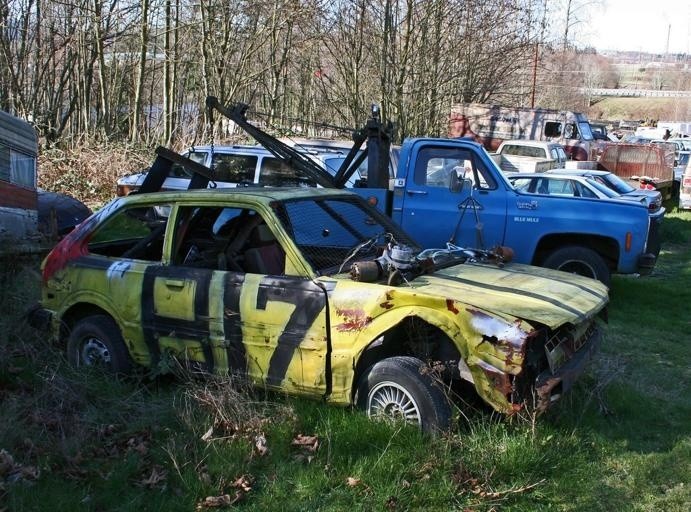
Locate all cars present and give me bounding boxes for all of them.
[37,183,95,246]
[39,185,610,445]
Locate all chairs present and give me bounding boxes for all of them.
[244,225,285,279]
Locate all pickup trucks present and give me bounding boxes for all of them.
[141,94,654,296]
